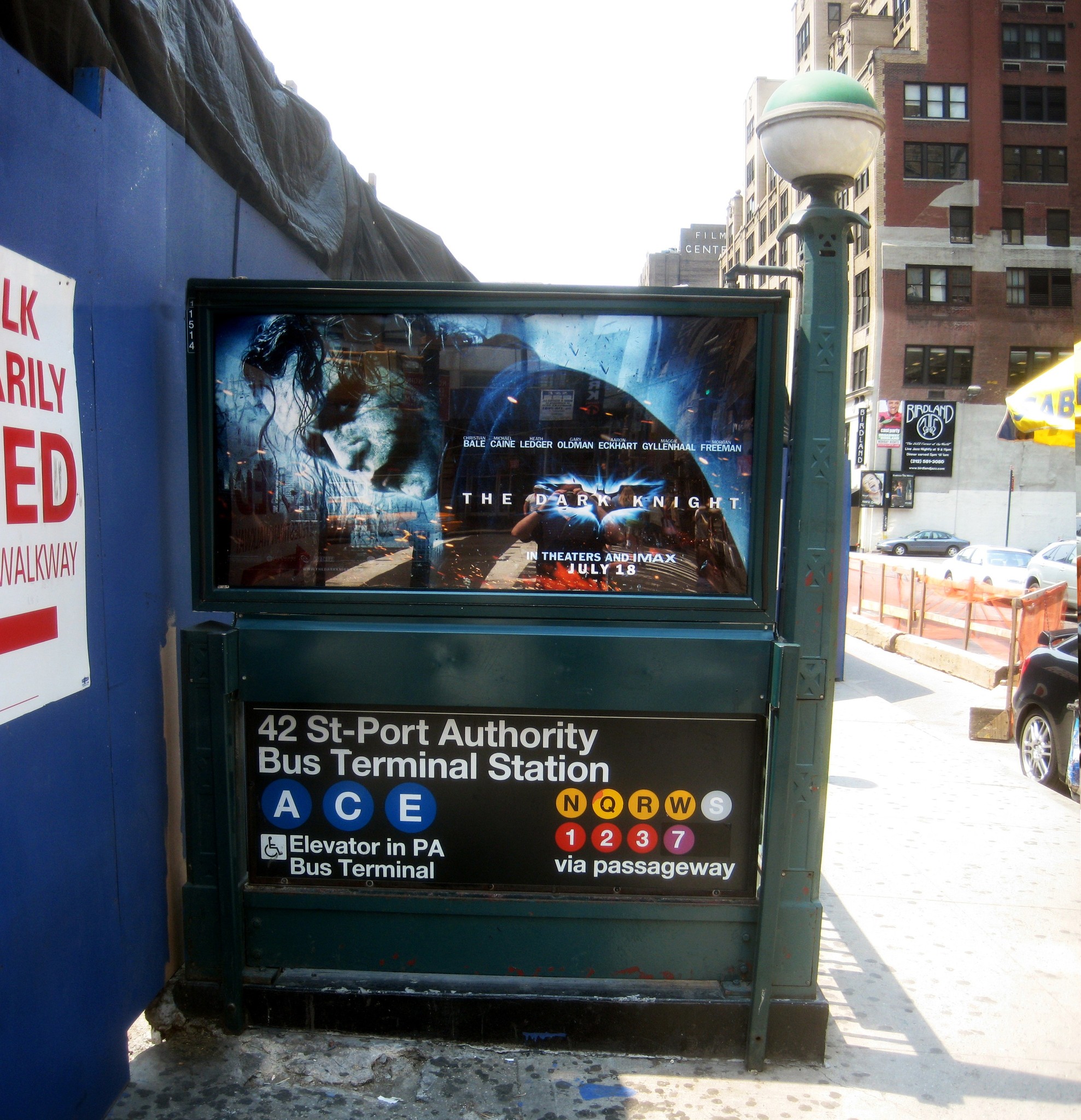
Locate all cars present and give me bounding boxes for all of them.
[875,529,971,558]
[938,545,1036,607]
[1024,539,1081,610]
[1011,627,1081,793]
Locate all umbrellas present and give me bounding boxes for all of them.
[1005,341,1081,447]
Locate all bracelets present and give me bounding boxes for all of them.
[535,509,544,516]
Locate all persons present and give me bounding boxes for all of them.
[877,400,902,433]
[891,480,903,499]
[861,472,883,506]
[241,315,746,598]
[510,480,713,578]
